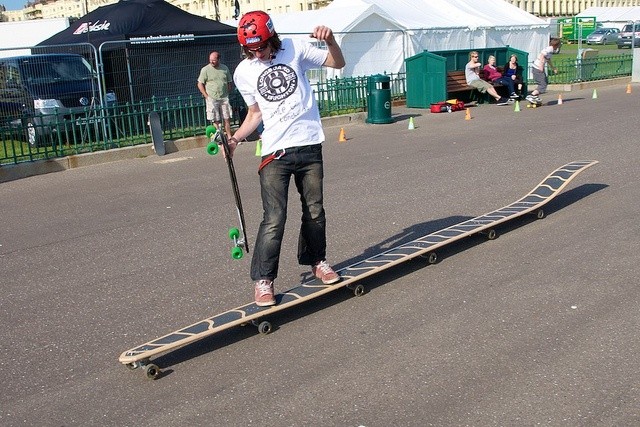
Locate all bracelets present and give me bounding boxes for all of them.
[230,137,238,144]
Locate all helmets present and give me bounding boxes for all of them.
[236,10,275,48]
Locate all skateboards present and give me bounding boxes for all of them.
[206,106,249,259]
[147,111,165,155]
[119,160,600,380]
[527,102,543,107]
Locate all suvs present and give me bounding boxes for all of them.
[587,27,620,44]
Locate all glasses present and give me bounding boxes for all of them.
[245,41,271,52]
[473,55,479,58]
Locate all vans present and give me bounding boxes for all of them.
[0,54,117,147]
[617,23,640,48]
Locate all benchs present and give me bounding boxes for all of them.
[447,66,505,101]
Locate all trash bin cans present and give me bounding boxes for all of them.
[365,74,394,123]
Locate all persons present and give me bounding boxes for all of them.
[221,10,345,306]
[525,38,561,104]
[465,51,511,106]
[503,54,523,101]
[483,55,520,99]
[197,52,242,148]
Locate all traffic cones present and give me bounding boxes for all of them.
[338,127,346,141]
[408,116,416,129]
[514,100,520,112]
[255,140,262,156]
[592,88,597,98]
[626,84,632,93]
[465,107,472,120]
[558,94,563,104]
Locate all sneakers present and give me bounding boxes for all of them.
[311,259,341,285]
[510,93,520,98]
[534,96,543,103]
[525,95,538,104]
[496,98,509,106]
[503,97,515,105]
[254,279,277,307]
